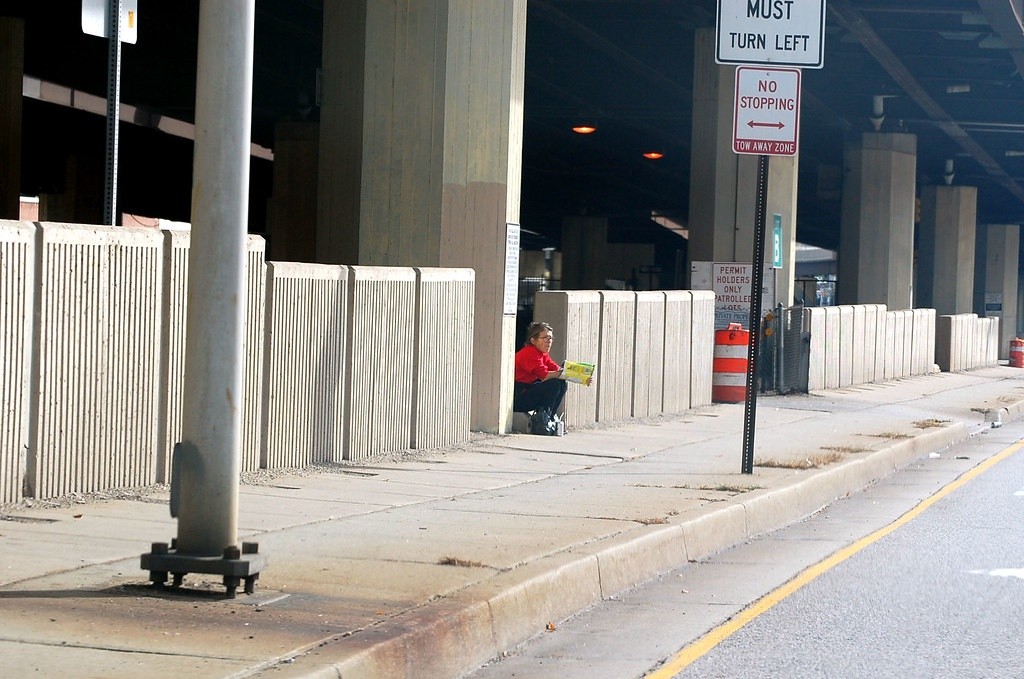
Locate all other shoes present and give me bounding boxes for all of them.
[530,415,556,436]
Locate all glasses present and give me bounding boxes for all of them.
[534,335,555,343]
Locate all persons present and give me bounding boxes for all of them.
[513,321,593,436]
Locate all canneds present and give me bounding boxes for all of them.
[556,420,564,436]
